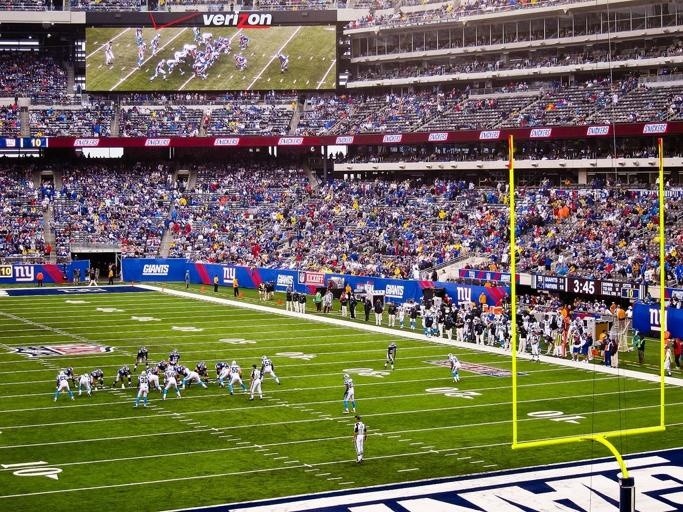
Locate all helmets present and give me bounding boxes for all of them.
[84,346,268,378]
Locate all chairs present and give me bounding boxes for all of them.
[0,0,683,292]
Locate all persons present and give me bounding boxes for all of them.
[353,416,367,464]
